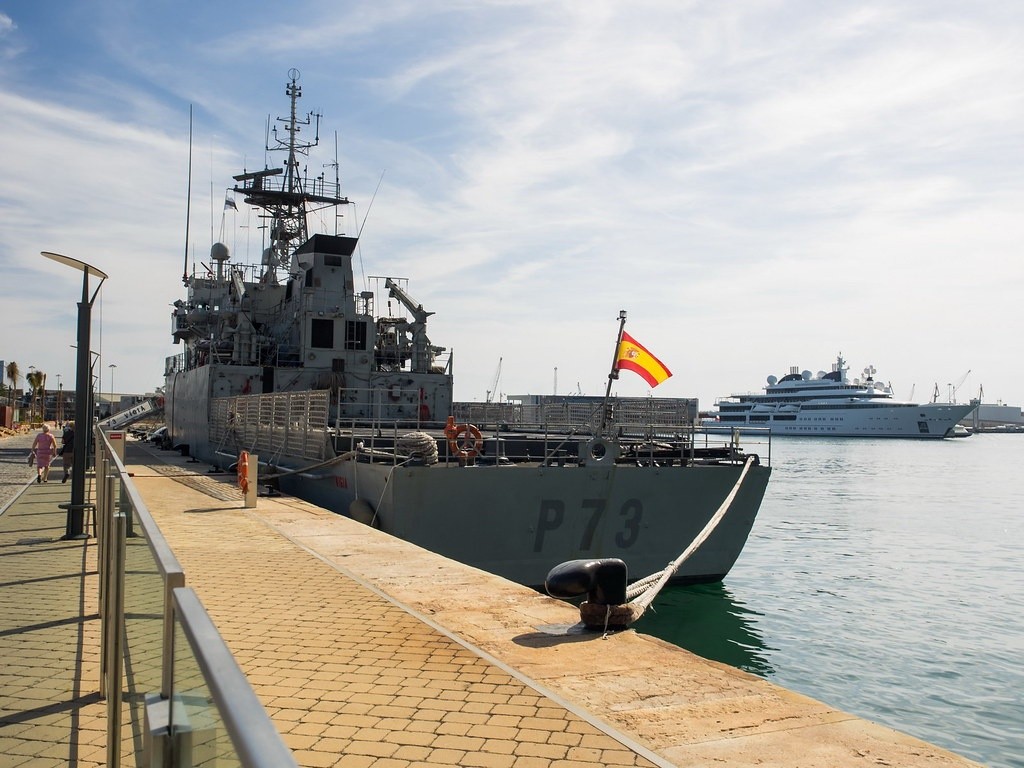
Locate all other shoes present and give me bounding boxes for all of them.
[62,473,70,483]
[44,480,47,483]
[37,474,41,483]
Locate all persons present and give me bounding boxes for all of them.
[61,422,75,483]
[31,424,57,484]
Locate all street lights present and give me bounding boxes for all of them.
[39,249,112,543]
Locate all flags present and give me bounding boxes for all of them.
[615,330,673,388]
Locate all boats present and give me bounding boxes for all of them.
[160,69,774,594]
[944,424,973,439]
[698,351,981,441]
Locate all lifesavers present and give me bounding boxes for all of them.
[449,425,484,458]
[585,437,611,463]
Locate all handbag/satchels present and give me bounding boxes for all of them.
[59,445,65,456]
